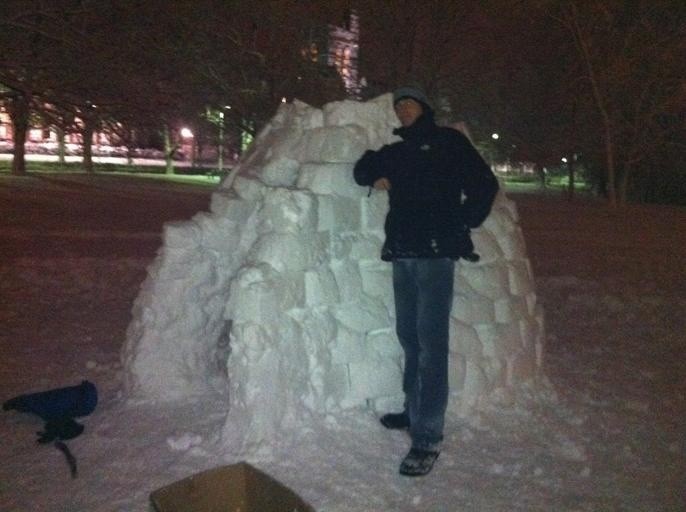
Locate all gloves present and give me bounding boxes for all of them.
[454,225,480,262]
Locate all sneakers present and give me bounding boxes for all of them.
[399,442,441,475]
[380,410,411,430]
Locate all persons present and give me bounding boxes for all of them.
[353,80,502,480]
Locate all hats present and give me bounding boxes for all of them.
[393,70,430,107]
